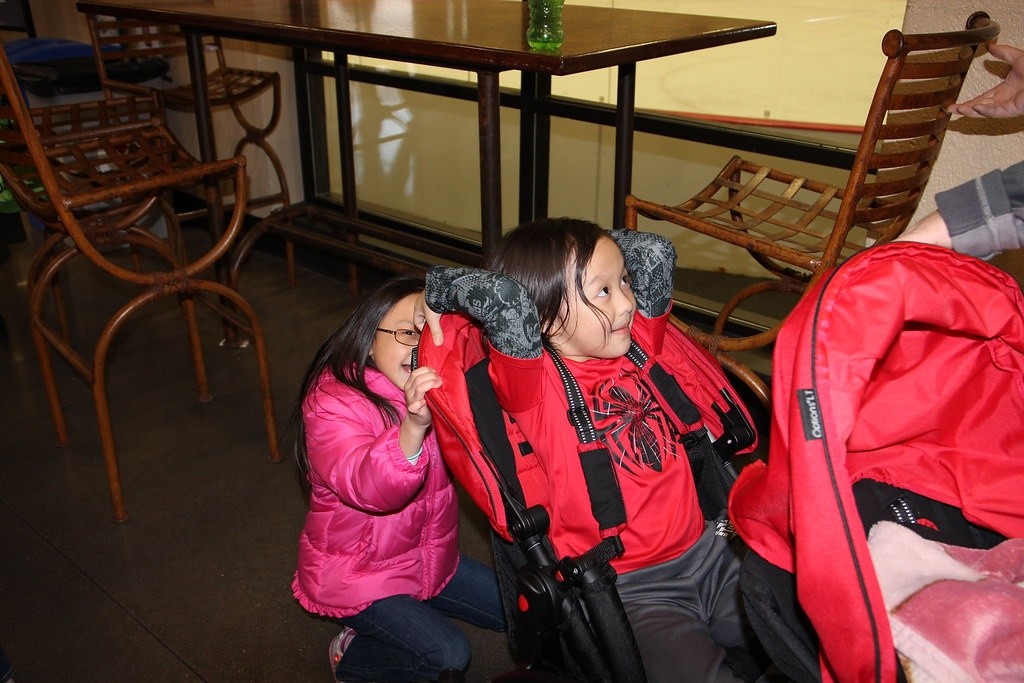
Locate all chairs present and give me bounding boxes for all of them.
[0,0,296,524]
[625,11,996,431]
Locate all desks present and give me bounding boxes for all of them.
[75,0,775,348]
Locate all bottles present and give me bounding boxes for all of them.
[525,0,566,51]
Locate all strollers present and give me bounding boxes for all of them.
[730,238,1024,683]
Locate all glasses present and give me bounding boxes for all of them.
[376,328,421,346]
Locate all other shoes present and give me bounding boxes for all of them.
[329,627,353,683]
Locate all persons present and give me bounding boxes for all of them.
[292,274,507,683]
[413,218,778,682]
[881,41,1023,258]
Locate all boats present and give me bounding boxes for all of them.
[412,310,758,683]
